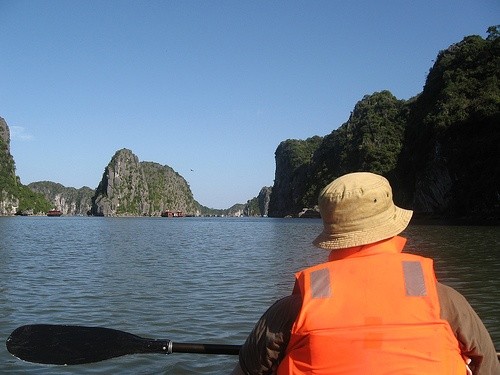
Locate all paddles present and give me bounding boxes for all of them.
[6,323,500,366]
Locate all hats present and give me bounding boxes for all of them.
[311,172,414,250]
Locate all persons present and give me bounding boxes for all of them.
[239,172,500,375]
